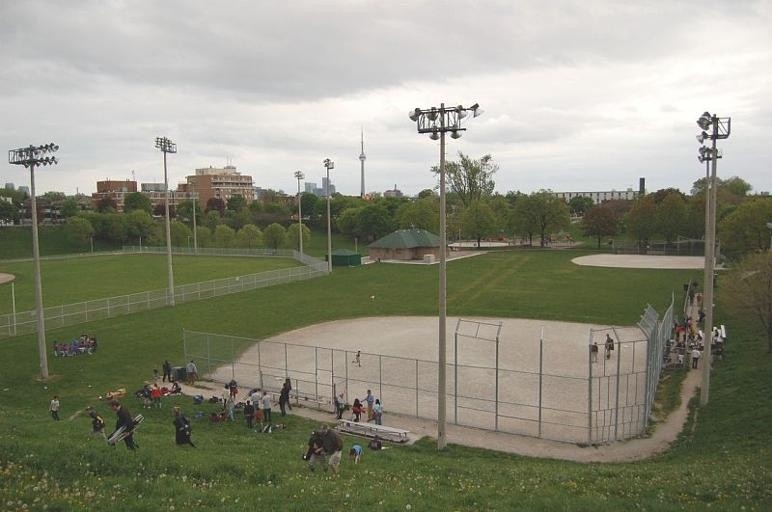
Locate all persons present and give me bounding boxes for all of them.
[85,405,107,444]
[667,280,726,370]
[140,360,384,476]
[603,333,612,360]
[351,351,362,367]
[50,332,98,360]
[591,341,598,363]
[48,395,60,422]
[106,398,136,454]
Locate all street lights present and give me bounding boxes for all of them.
[695,110,732,410]
[293,170,307,263]
[324,157,335,275]
[188,190,202,255]
[9,141,59,383]
[155,137,180,306]
[410,102,485,453]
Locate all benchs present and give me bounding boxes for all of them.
[337,419,409,442]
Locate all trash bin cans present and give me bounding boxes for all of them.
[170,366,182,382]
